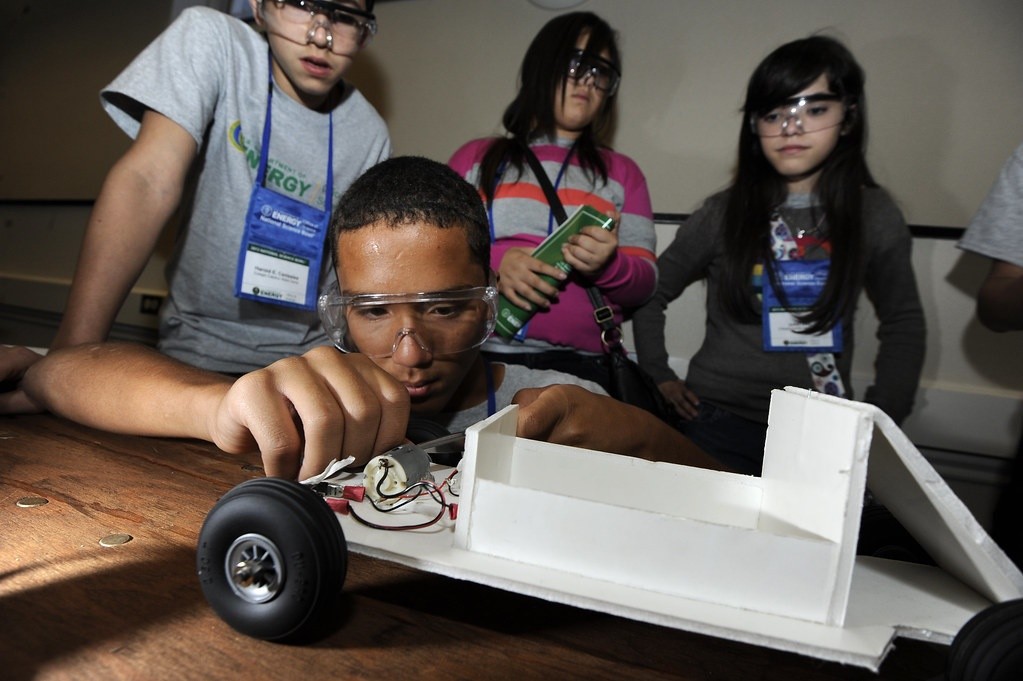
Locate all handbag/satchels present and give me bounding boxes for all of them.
[609,340,677,429]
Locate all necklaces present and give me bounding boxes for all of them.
[775,193,829,239]
[480,357,496,420]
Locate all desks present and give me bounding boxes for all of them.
[0,412,938,681]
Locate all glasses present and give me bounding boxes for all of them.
[750,92,855,137]
[317,267,499,356]
[566,47,622,95]
[256,0,379,55]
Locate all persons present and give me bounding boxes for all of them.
[631,34,930,482]
[956,144,1022,332]
[21,155,729,473]
[442,11,660,389]
[1,0,394,419]
